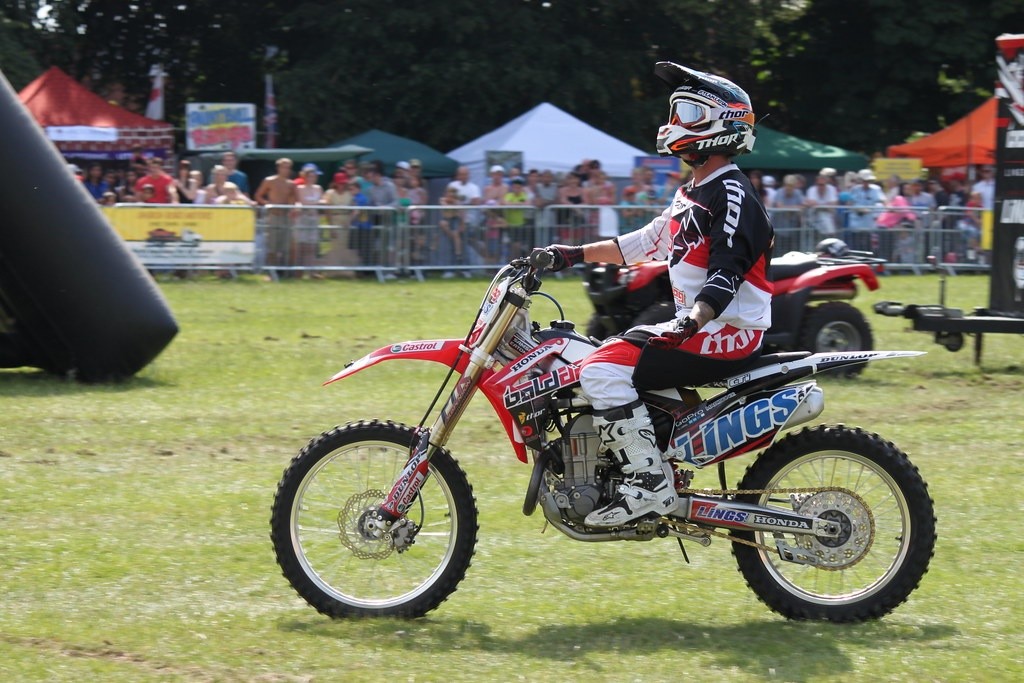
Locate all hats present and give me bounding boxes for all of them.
[490,164,506,174]
[396,160,410,170]
[761,166,877,184]
[410,158,421,168]
[301,163,324,175]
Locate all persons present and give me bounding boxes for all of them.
[438,159,684,279]
[750,167,995,267]
[551,62,776,528]
[70,144,428,281]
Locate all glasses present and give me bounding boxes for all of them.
[669,99,711,127]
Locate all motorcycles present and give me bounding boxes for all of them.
[572,237,884,383]
[270,243,939,626]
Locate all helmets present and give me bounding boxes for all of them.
[654,60,754,168]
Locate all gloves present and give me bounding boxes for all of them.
[647,316,698,351]
[542,243,584,273]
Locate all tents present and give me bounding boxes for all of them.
[888,96,996,166]
[729,124,869,171]
[337,130,461,177]
[18,65,175,179]
[446,102,651,199]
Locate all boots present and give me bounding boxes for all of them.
[584,400,680,528]
